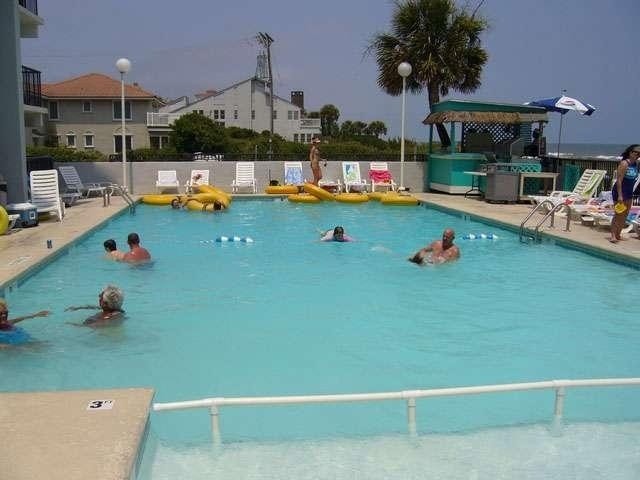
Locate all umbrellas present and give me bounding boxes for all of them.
[525,88,596,173]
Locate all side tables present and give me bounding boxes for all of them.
[58,192,82,207]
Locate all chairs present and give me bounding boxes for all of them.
[184,169,209,193]
[29,169,66,222]
[527,168,608,216]
[368,161,397,192]
[342,161,371,193]
[318,161,343,196]
[156,170,179,194]
[58,166,114,199]
[233,162,257,194]
[285,161,302,185]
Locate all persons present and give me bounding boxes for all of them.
[102,238,125,263]
[308,136,328,188]
[65,286,126,329]
[609,145,640,242]
[170,199,182,209]
[317,226,353,243]
[120,235,151,265]
[0,298,53,354]
[524,127,543,156]
[192,174,203,185]
[410,230,458,266]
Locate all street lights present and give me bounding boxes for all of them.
[396,61,413,189]
[114,56,132,187]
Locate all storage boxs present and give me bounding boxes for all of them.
[5,203,38,228]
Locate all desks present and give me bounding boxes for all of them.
[464,171,488,198]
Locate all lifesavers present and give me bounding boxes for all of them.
[143,184,231,211]
[0,206,8,234]
[265,183,419,204]
